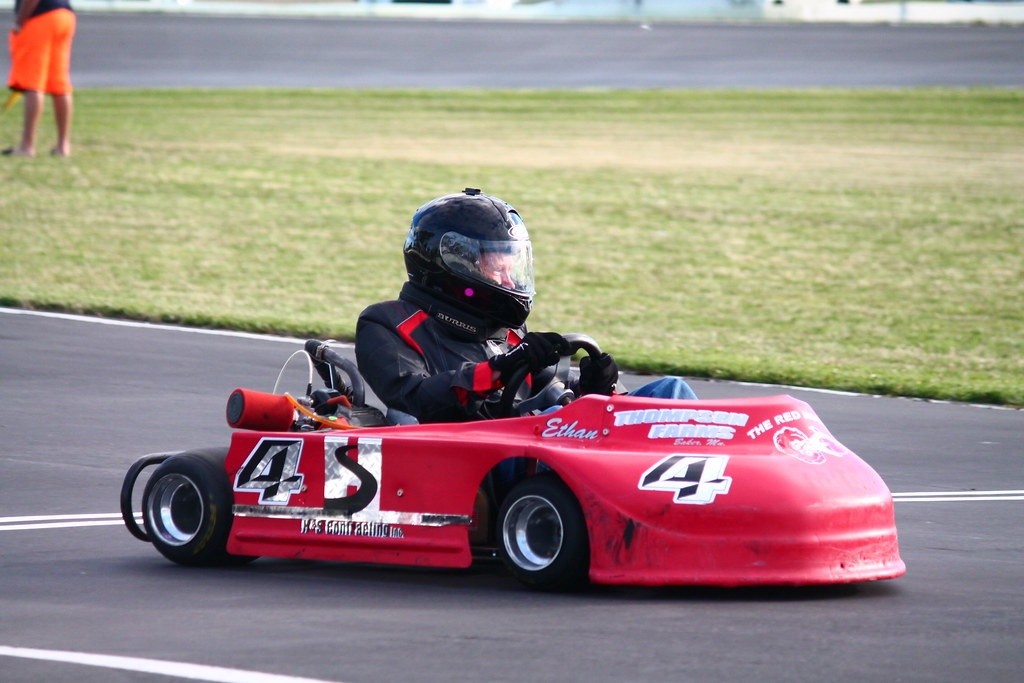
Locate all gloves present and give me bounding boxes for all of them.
[489,330,570,385]
[578,351,619,397]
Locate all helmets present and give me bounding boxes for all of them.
[403,188,534,329]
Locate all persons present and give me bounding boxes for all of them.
[4,0,77,159]
[354,188,699,428]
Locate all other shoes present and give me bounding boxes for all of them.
[50,148,64,157]
[1,147,33,159]
